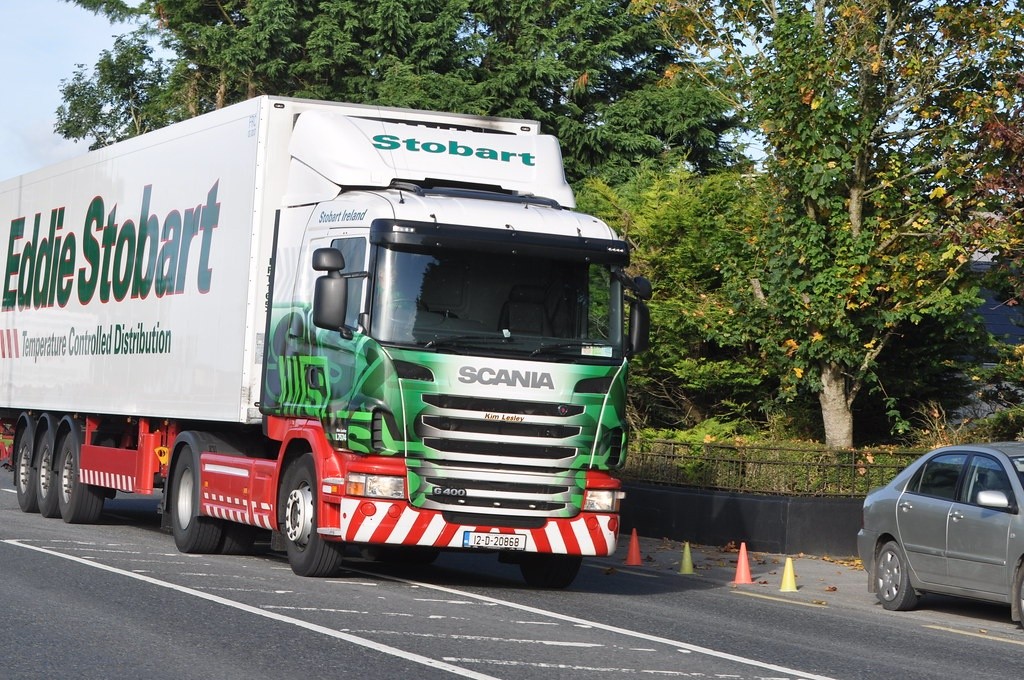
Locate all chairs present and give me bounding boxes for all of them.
[984,469,1004,491]
[497,285,554,337]
[929,468,959,499]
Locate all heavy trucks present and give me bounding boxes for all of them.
[0,93,651,578]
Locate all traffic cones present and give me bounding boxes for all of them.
[729,542,758,585]
[778,556,799,592]
[676,541,697,575]
[623,528,645,567]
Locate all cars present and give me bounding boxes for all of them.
[856,441,1024,633]
